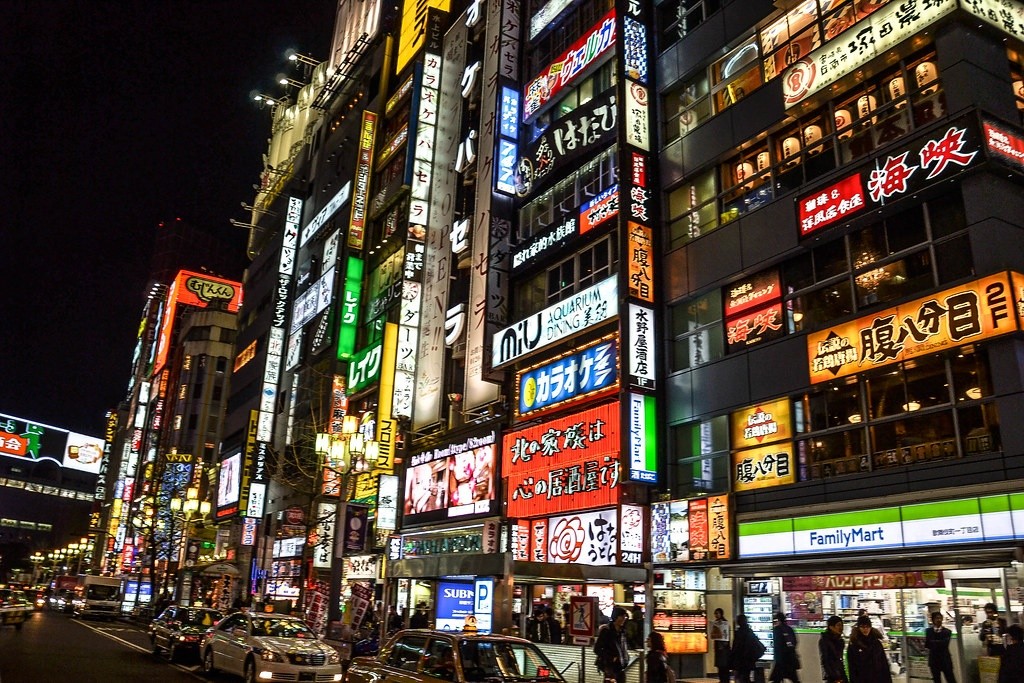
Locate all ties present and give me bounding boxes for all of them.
[935,629,939,632]
[537,624,541,641]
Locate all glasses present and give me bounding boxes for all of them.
[713,612,719,615]
[986,610,995,614]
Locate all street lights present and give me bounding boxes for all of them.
[73,538,94,575]
[132,509,166,605]
[314,416,380,635]
[170,487,211,568]
[61,544,79,570]
[48,549,65,571]
[30,552,45,578]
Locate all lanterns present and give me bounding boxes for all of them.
[888,61,937,109]
[782,137,800,166]
[835,110,852,140]
[857,95,877,126]
[757,152,771,181]
[737,163,754,190]
[804,125,823,155]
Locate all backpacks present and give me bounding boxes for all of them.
[656,654,675,683]
[629,618,644,644]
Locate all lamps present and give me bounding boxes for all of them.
[847,414,862,423]
[903,402,922,411]
[856,261,885,289]
[250,48,320,108]
[396,414,426,435]
[230,164,306,232]
[149,282,168,300]
[448,393,484,417]
[966,387,982,400]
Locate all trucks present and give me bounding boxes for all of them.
[73,574,121,621]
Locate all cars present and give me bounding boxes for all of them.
[345,628,568,683]
[148,604,224,665]
[198,611,343,683]
[0,582,80,631]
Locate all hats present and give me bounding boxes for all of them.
[854,615,873,626]
[1003,625,1024,641]
[772,613,785,621]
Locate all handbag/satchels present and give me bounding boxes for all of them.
[594,656,611,676]
[793,653,800,670]
[710,625,721,639]
[748,630,767,665]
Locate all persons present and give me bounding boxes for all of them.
[847,615,892,683]
[818,616,848,683]
[713,608,730,683]
[768,613,800,683]
[729,615,755,683]
[962,616,973,626]
[374,601,429,629]
[978,603,1007,641]
[232,594,274,612]
[526,604,644,649]
[645,632,668,683]
[925,612,956,683]
[987,625,1024,683]
[593,608,629,683]
